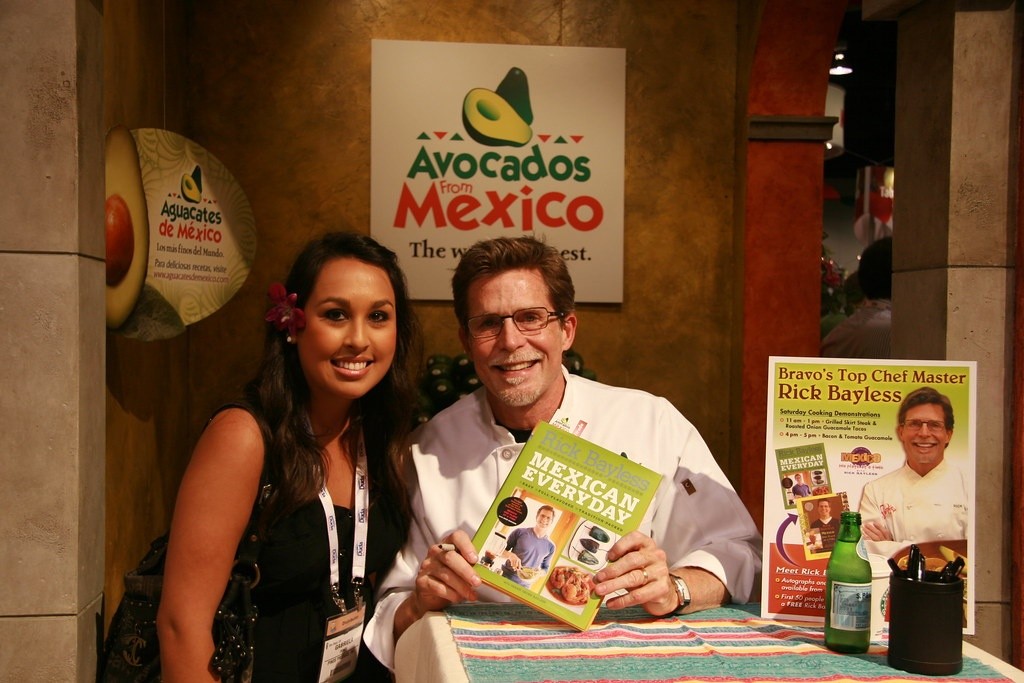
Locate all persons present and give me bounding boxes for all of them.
[859,386,969,562]
[100,228,426,683]
[820,237,893,358]
[501,506,556,590]
[363,237,764,674]
[810,499,840,553]
[792,473,810,500]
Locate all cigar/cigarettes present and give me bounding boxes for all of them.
[439,544,456,550]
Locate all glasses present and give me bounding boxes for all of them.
[901,420,946,432]
[466,306,565,339]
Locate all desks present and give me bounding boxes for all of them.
[393,599,1023,683]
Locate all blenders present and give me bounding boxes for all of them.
[480,532,507,568]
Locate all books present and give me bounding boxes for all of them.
[466,420,664,631]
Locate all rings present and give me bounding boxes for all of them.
[641,569,648,585]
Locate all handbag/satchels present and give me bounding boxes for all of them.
[96,530,264,683]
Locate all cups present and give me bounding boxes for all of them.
[887,569,964,676]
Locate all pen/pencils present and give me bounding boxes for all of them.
[937,557,965,583]
[888,557,904,577]
[438,543,457,552]
[907,544,925,582]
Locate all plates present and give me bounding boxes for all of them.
[569,520,617,572]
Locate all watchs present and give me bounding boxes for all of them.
[666,573,691,618]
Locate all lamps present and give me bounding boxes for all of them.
[830,51,853,74]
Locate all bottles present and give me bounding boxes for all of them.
[824,511,872,654]
